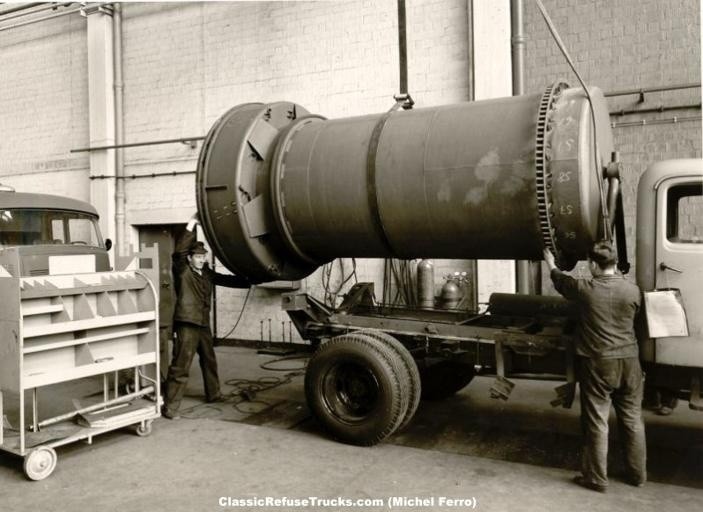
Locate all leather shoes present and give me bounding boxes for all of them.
[163,409,180,420]
[205,393,230,403]
[619,475,645,487]
[573,476,609,493]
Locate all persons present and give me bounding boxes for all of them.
[543,240,649,493]
[158,212,254,421]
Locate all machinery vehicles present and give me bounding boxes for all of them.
[283,158,703,445]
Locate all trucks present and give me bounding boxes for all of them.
[2,191,110,274]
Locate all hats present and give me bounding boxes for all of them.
[187,241,208,254]
